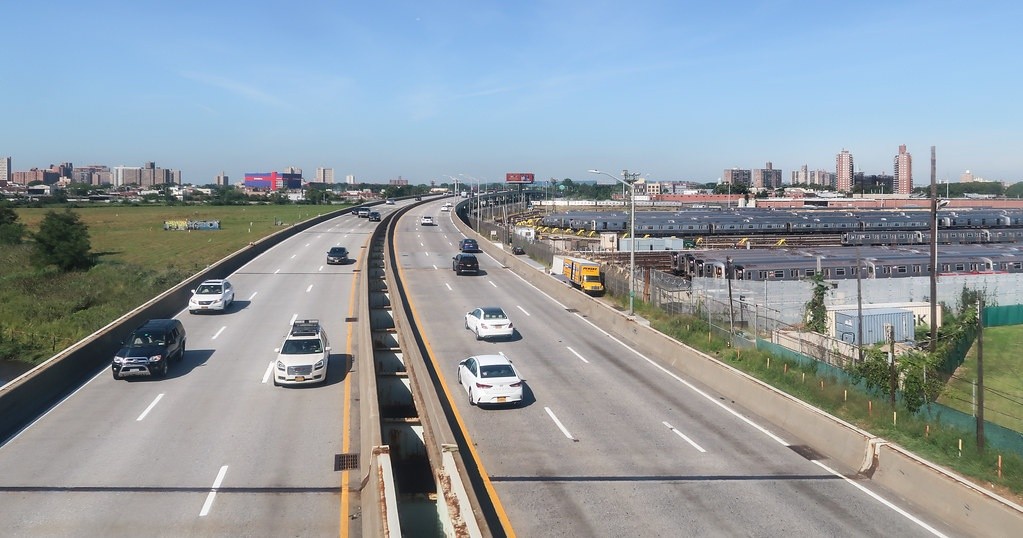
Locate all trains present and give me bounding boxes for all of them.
[531,202,1023,288]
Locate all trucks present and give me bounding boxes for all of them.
[550,255,604,294]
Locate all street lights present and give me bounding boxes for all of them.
[586,169,634,315]
[442,174,487,233]
[931,145,950,350]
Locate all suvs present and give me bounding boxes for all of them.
[112,318,186,379]
[273,318,332,387]
[512,245,524,255]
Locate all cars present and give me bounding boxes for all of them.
[352,207,381,221]
[327,246,349,264]
[465,304,514,341]
[386,198,394,204]
[441,201,452,210]
[458,239,478,252]
[189,279,235,314]
[452,253,480,275]
[457,354,523,407]
[420,215,433,226]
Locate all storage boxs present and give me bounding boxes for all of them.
[807,302,942,345]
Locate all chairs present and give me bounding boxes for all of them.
[492,315,496,318]
[287,345,297,353]
[201,288,209,293]
[486,314,489,317]
[499,315,502,318]
[134,337,152,344]
